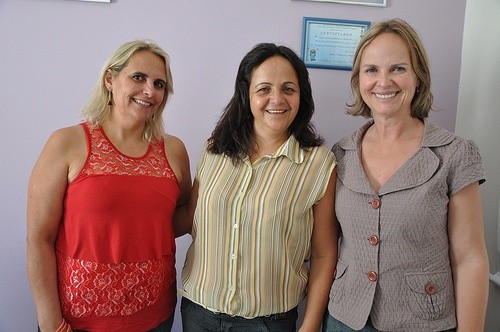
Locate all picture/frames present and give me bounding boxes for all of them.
[298,16,373,74]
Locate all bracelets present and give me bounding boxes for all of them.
[56,319,72,332]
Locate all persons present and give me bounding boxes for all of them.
[319,18,490,332]
[27,39,193,332]
[172,43,339,332]
